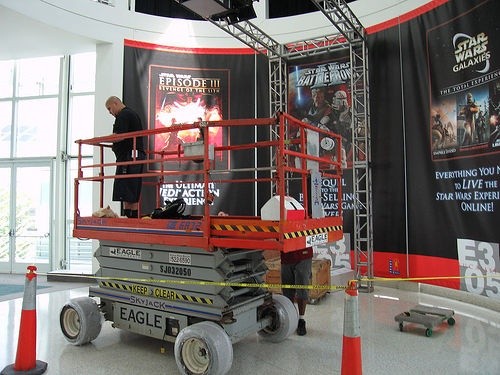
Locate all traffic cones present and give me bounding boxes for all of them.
[0,264,48,375]
[339,277,368,375]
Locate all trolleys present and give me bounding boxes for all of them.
[394,304,456,339]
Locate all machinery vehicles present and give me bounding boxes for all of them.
[59,111,345,375]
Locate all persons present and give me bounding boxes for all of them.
[279,246,314,336]
[103,95,146,219]
